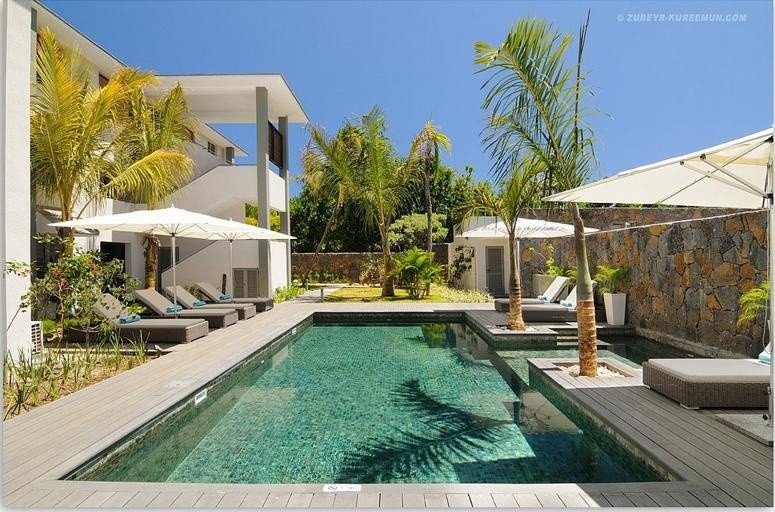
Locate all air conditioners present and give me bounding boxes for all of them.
[31,321,44,355]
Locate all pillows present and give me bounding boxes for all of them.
[758,341,771,365]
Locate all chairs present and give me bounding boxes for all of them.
[640,339,771,411]
[69,280,274,344]
[494,274,607,324]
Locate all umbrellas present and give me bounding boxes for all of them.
[178,216,300,298]
[47,203,243,313]
[541,121,773,431]
[453,213,599,298]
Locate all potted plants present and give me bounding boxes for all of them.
[593,263,632,327]
